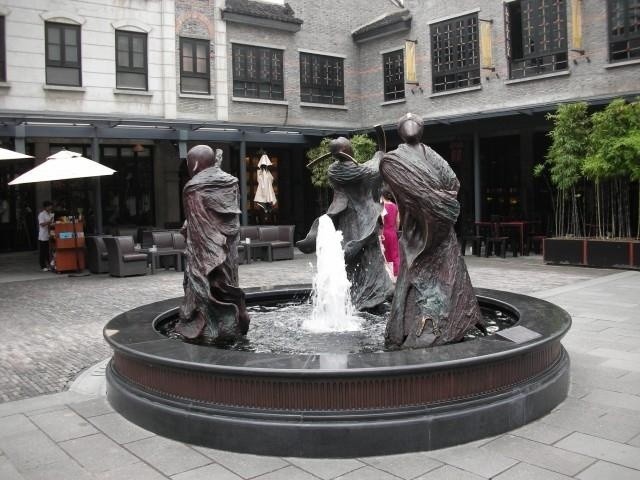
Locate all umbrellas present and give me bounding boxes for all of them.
[0,147,36,160]
[7,148,118,274]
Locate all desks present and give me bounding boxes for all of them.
[473,221,541,256]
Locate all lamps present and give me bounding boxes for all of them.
[132,143,144,152]
[256,144,267,155]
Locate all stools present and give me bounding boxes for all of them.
[525,235,547,255]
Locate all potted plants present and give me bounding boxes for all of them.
[533,95,640,270]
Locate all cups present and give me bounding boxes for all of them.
[246,238,251,244]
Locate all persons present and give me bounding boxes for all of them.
[380,189,400,280]
[176,145,250,345]
[37,201,56,272]
[297,137,395,316]
[380,113,488,350]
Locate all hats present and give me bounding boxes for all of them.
[43,201,54,207]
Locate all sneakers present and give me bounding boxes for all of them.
[40,268,51,272]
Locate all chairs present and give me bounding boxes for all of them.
[484,214,517,258]
[86,224,297,278]
[461,221,486,257]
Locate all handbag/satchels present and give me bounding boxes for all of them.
[385,262,394,277]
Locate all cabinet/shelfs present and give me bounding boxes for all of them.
[47,222,86,272]
